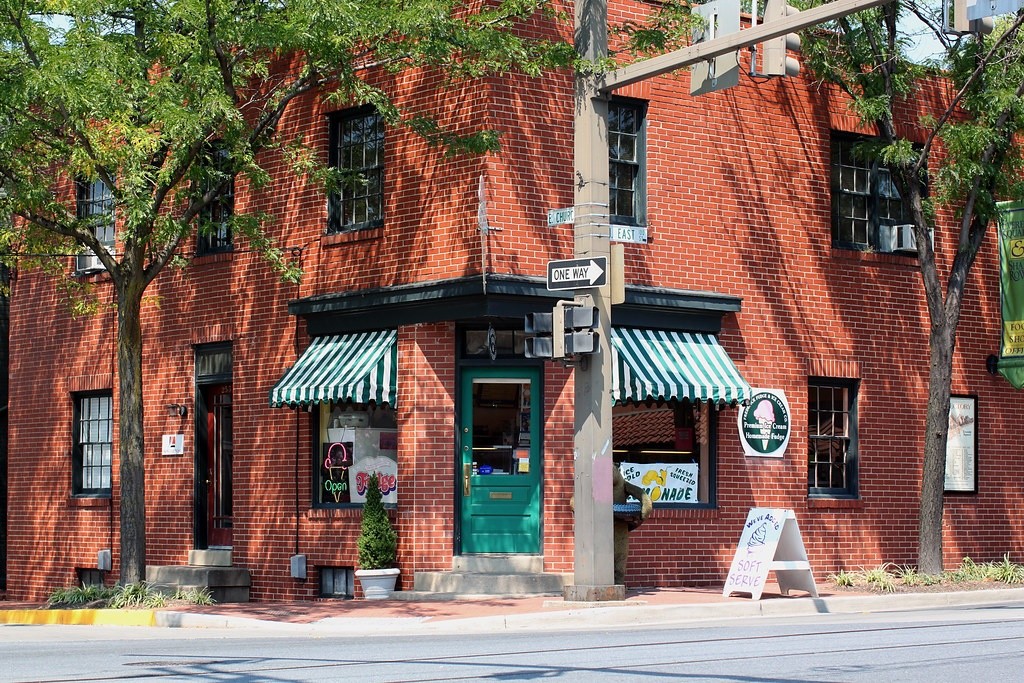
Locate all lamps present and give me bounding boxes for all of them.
[167,404,187,417]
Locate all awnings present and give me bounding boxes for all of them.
[268,330,398,410]
[610,327,753,407]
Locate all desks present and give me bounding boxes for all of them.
[474,448,512,475]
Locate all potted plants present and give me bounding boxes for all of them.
[356,470,400,600]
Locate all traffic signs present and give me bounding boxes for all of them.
[547,256,607,292]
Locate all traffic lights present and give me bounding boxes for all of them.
[953,0,995,36]
[524,312,553,359]
[763,0,801,79]
[563,307,600,354]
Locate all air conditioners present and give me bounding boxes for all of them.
[891,224,935,253]
[76,245,115,272]
